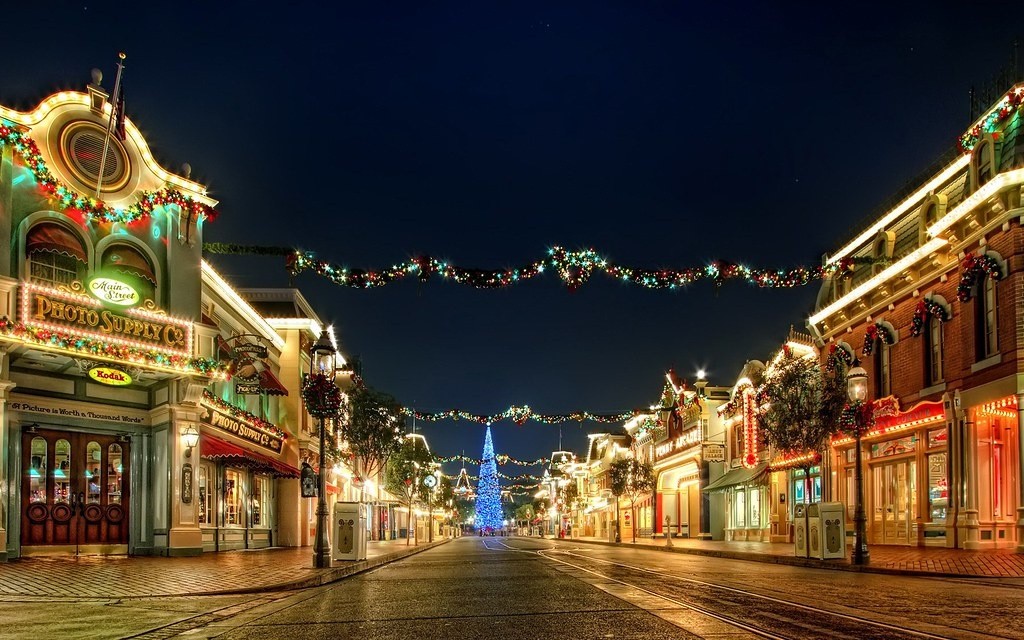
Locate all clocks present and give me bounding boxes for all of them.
[423,475,438,488]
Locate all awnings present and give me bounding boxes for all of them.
[199,432,300,480]
[259,359,289,396]
[583,493,653,515]
[325,481,340,494]
[701,463,768,492]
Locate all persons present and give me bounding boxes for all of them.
[234,360,267,378]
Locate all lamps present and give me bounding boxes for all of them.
[181,422,200,457]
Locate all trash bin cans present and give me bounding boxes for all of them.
[518,525,539,537]
[554,525,559,538]
[451,527,463,538]
[417,520,429,543]
[443,525,450,539]
[609,520,621,541]
[571,524,579,539]
[792,501,846,559]
[333,501,367,561]
